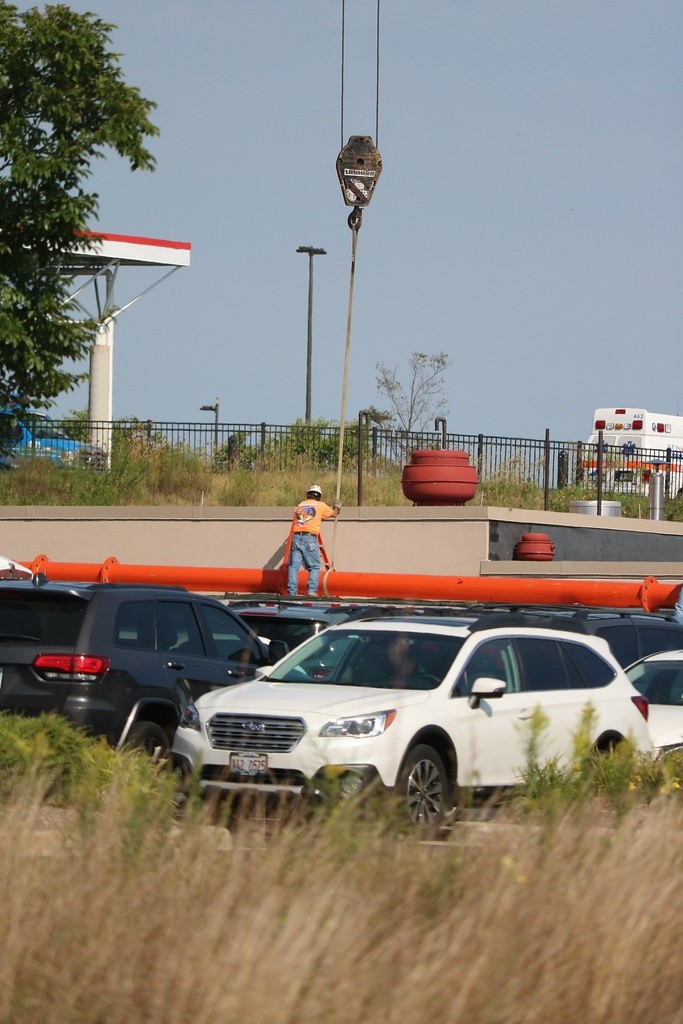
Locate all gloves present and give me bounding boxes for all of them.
[333,499,343,510]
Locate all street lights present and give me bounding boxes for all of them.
[200,401,219,457]
[297,245,329,430]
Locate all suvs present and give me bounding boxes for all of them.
[0,571,290,786]
[225,597,367,683]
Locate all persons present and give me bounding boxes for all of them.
[287,485,341,596]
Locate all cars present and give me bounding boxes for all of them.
[620,650,683,759]
[177,616,653,835]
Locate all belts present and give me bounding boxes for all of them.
[294,532,317,537]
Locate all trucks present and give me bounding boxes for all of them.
[0,394,109,476]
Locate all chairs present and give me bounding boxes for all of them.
[138,619,180,653]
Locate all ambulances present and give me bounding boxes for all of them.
[581,407,683,499]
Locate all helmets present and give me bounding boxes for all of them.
[306,485,322,495]
